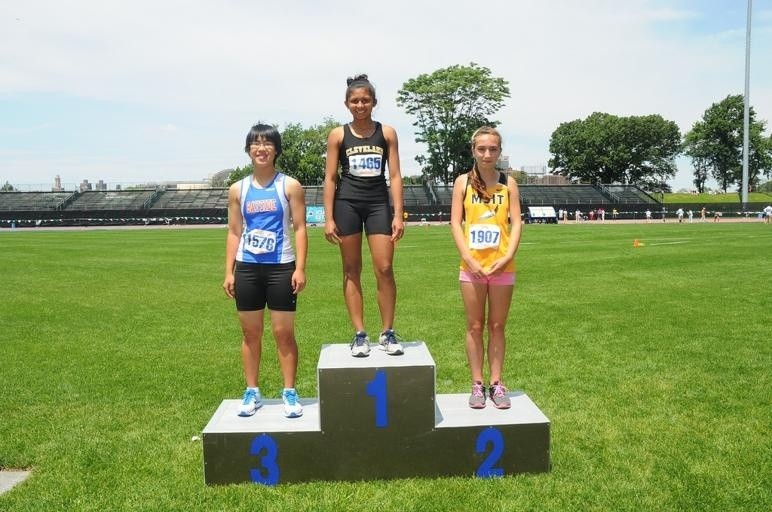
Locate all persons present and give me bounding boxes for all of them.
[222,124,308,418]
[641,203,724,223]
[555,204,619,222]
[323,75,405,357]
[449,126,523,409]
[740,203,772,224]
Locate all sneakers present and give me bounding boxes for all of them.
[349,330,371,357]
[378,328,404,355]
[468,380,486,408]
[282,387,303,418]
[488,380,511,409]
[236,386,263,417]
